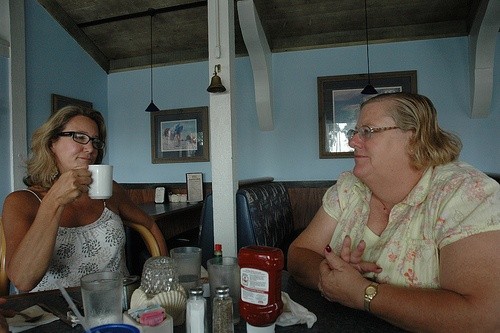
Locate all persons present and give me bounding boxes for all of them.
[288,93,500,333]
[3,106,169,293]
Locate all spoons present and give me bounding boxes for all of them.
[0,304,44,323]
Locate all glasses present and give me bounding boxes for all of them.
[346,126,401,141]
[56,131,105,150]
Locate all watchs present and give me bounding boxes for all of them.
[364,281,377,313]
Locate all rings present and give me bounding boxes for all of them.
[321,291,324,298]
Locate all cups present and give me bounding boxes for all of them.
[80,271,123,328]
[87,164,114,200]
[206,256,241,324]
[170,246,203,297]
[87,323,141,333]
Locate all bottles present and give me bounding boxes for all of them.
[237,245,285,332]
[185,287,209,333]
[214,244,222,265]
[211,286,234,333]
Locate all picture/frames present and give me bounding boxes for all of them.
[150,106,210,163]
[317,70,418,157]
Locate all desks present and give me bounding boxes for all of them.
[122,200,203,239]
[0,270,414,333]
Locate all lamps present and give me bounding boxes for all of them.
[144,8,160,112]
[207,64,226,93]
[361,0,378,95]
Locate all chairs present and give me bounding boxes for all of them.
[0,216,158,297]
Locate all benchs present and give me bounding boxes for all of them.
[166,192,214,266]
[237,182,303,275]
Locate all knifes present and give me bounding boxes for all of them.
[38,301,77,327]
